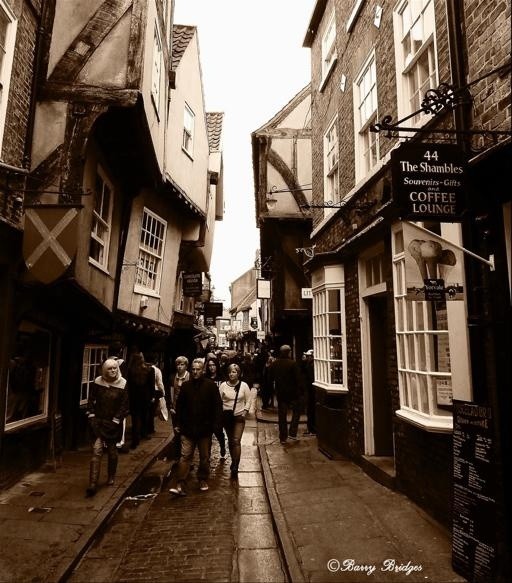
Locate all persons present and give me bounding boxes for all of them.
[204,356,226,460]
[168,358,224,492]
[84,358,128,496]
[219,362,253,479]
[102,342,130,454]
[167,354,196,467]
[128,349,155,450]
[148,356,167,435]
[300,348,317,436]
[272,342,302,444]
[205,346,276,410]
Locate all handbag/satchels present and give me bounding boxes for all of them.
[223,410,234,428]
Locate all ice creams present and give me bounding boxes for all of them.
[408,239,429,283]
[437,250,456,285]
[420,240,442,280]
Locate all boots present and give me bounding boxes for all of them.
[86,461,101,495]
[107,455,118,485]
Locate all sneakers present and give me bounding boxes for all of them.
[221,446,225,457]
[169,487,186,496]
[199,480,209,491]
[230,463,238,479]
